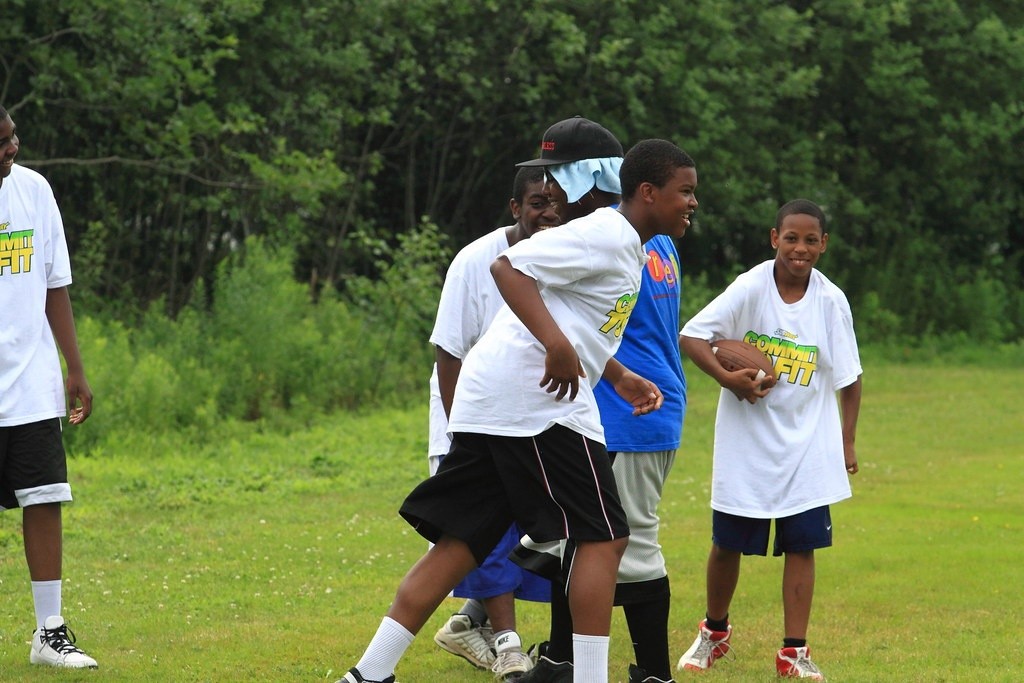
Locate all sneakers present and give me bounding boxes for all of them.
[433,612,497,671]
[335,667,395,683]
[627,662,676,683]
[677,617,733,670]
[505,641,574,683]
[774,643,824,679]
[490,630,537,682]
[30,615,100,670]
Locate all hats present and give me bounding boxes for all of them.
[515,115,625,168]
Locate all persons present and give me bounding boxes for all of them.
[424,156,567,682]
[509,114,688,681]
[332,138,700,682]
[678,197,863,682]
[0,102,101,673]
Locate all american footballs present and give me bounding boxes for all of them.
[709,339,777,390]
